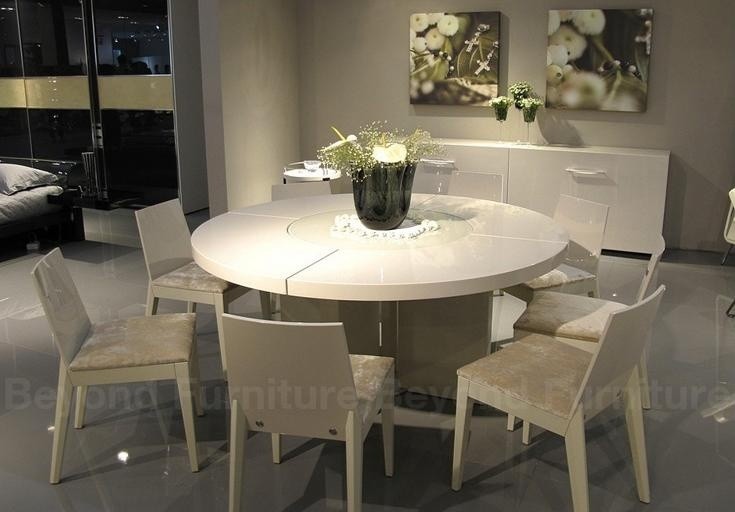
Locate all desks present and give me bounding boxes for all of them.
[282,168,341,184]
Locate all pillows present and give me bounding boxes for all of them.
[1,162,58,197]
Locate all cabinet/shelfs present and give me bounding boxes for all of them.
[395,136,670,262]
[0,0,208,216]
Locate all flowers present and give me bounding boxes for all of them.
[316,116,447,181]
[488,79,544,123]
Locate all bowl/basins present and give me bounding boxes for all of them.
[304,160,321,172]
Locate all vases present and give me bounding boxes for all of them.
[351,161,417,231]
[513,93,527,144]
[494,105,508,144]
[522,107,537,145]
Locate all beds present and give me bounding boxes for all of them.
[0,155,84,261]
